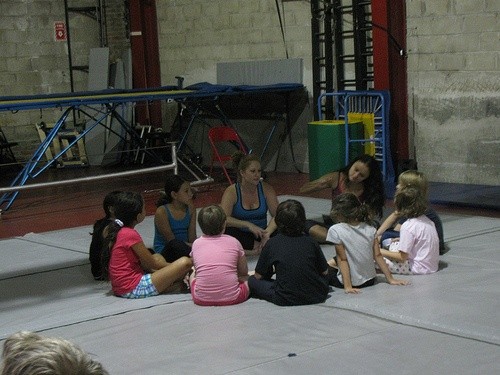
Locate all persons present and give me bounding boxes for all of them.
[0,329,108,375]
[89,156,444,306]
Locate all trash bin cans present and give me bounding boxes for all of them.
[304,118,368,185]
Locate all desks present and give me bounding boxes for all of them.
[0,83,304,216]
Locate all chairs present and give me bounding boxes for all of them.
[207,126,248,186]
